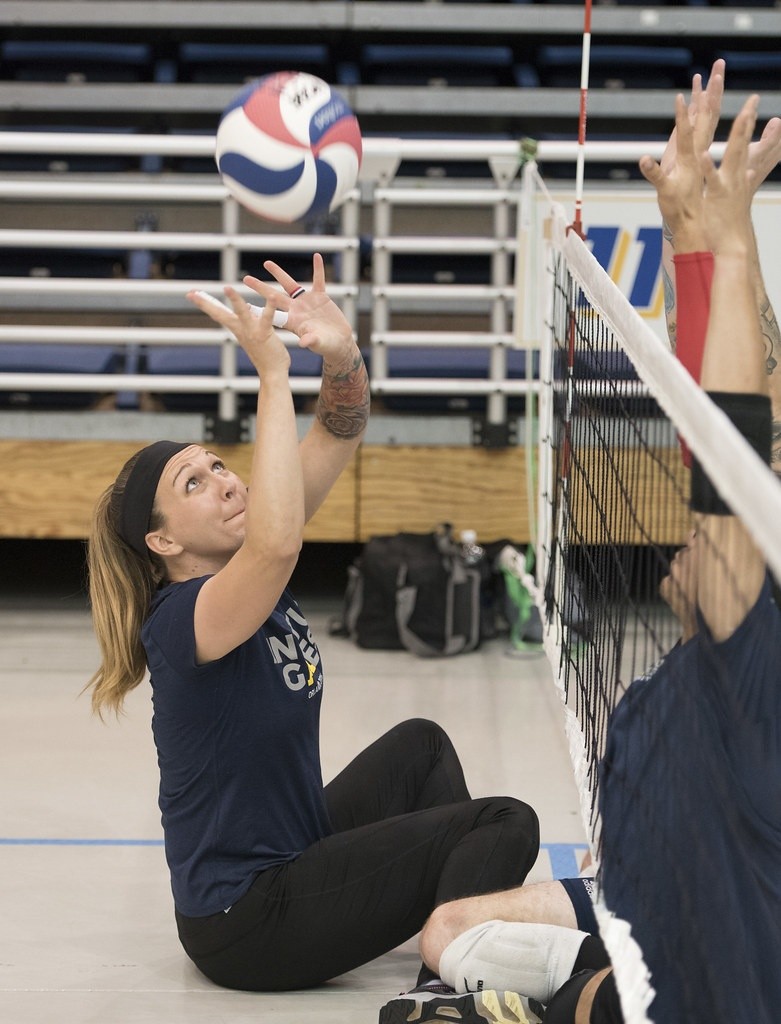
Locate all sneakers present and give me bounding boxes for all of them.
[379,979,547,1024]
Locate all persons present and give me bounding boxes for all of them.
[80,250,546,1023]
[418,59,781,1024]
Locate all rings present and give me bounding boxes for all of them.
[289,285,305,299]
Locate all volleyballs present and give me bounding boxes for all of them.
[215,67,362,224]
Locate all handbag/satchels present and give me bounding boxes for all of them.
[328,522,498,658]
